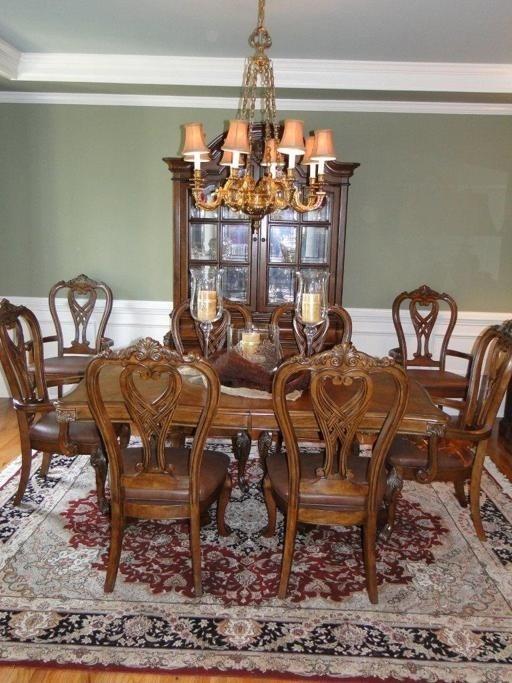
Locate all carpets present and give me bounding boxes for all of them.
[0,436,512,683]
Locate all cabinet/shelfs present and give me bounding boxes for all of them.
[162,121,360,360]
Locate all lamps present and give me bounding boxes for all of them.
[181,0,337,230]
